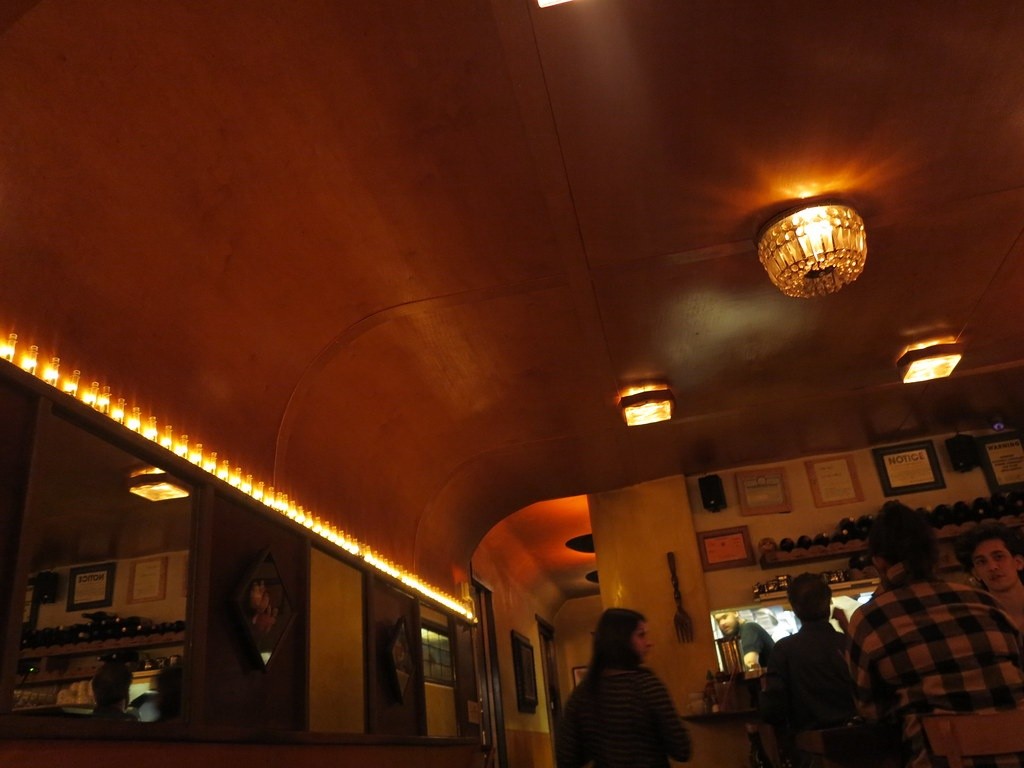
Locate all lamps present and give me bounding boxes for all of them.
[897,345,964,386]
[756,197,867,298]
[616,379,673,429]
[120,463,192,507]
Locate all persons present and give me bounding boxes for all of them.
[712,498,1024,768]
[88,663,140,717]
[556,606,692,767]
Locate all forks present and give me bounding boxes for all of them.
[667,552,694,644]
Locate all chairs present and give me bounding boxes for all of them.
[917,703,1024,768]
[793,715,898,768]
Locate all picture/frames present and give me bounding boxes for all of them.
[386,615,417,704]
[512,628,539,715]
[127,554,167,606]
[231,547,300,677]
[66,563,113,612]
[572,666,587,688]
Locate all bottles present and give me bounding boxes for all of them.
[750,486,1024,603]
[18,610,186,677]
[703,666,737,714]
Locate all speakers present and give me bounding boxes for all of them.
[698,474,727,512]
[945,433,983,473]
